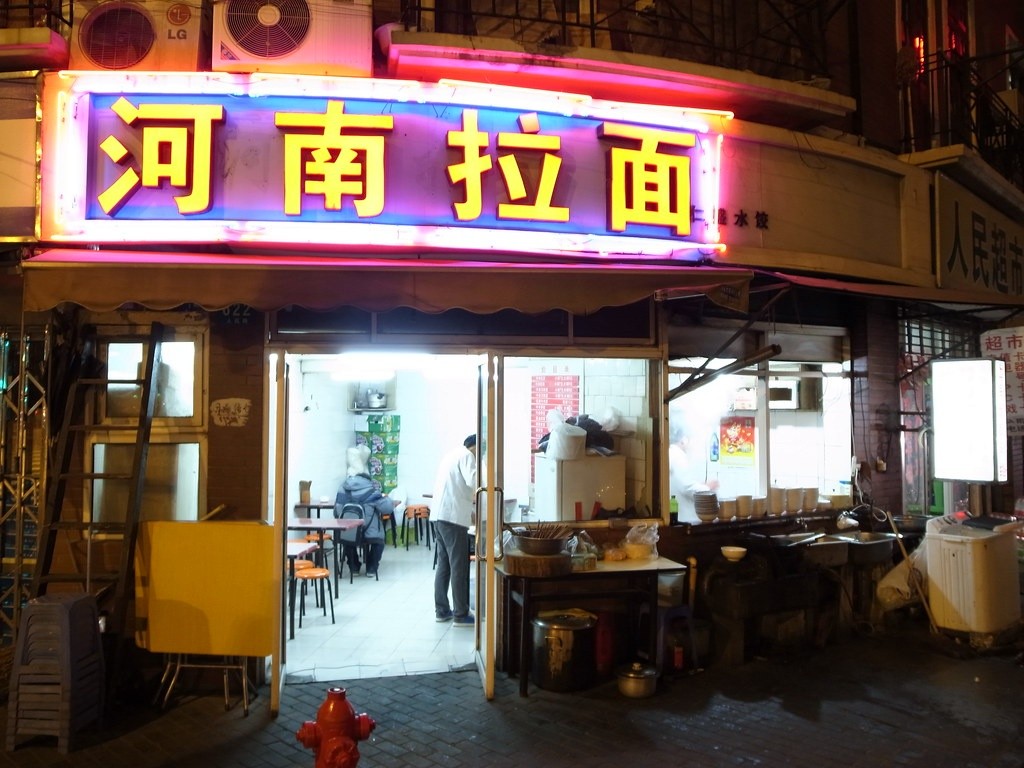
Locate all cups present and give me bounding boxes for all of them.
[301,490,310,504]
[353,401,357,408]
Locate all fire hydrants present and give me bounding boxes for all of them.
[297,687,376,768]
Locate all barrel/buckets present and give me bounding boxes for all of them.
[529,615,598,693]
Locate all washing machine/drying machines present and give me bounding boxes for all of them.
[923,510,1023,632]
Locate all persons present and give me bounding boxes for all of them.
[428,434,487,626]
[669,425,719,522]
[333,473,394,576]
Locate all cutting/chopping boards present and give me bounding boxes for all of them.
[503,551,573,576]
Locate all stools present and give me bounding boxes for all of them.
[6,592,106,754]
[286,533,335,627]
[400,504,436,551]
[383,515,397,549]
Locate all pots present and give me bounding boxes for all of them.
[367,391,387,408]
[615,661,660,699]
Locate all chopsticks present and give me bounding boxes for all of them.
[299,480,312,490]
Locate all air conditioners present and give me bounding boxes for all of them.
[212,1,374,79]
[68,0,203,72]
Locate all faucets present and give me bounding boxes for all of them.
[796,515,807,531]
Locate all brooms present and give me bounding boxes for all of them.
[886,510,965,660]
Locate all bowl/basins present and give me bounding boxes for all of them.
[720,546,747,561]
[666,618,711,658]
[517,530,572,555]
[692,488,832,523]
[892,513,934,531]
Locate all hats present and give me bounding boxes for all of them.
[464,434,476,448]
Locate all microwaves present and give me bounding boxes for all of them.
[767,380,801,411]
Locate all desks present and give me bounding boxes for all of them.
[287,542,319,639]
[287,517,364,599]
[494,554,687,697]
[135,519,281,716]
[294,499,402,546]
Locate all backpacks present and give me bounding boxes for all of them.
[337,488,378,545]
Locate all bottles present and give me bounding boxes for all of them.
[670,495,678,525]
[710,434,719,463]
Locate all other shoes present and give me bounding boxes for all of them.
[435,610,453,622]
[454,615,475,627]
[353,562,362,576]
[366,563,380,577]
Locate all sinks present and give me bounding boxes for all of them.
[770,534,848,566]
[841,531,903,562]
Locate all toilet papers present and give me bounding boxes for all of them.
[547,424,587,460]
